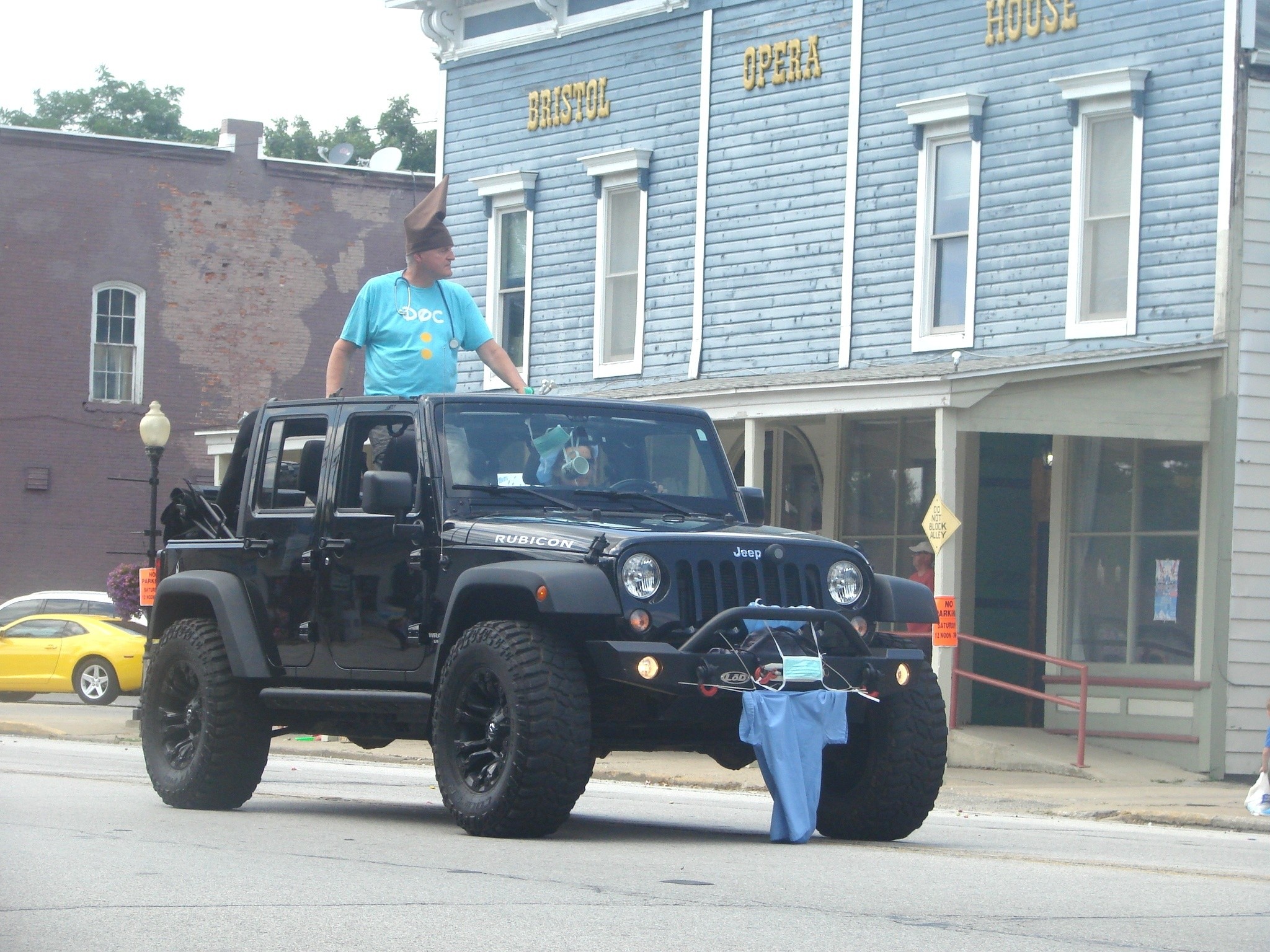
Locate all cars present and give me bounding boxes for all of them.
[0,589,125,618]
[0,610,148,703]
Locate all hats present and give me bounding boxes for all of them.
[909,541,933,554]
[545,426,597,446]
[403,174,453,255]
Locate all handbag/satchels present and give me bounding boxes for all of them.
[1244,771,1270,817]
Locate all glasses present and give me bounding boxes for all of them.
[914,552,927,556]
[563,458,594,466]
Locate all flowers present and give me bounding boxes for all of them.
[105,558,149,622]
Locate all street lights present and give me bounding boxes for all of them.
[136,399,172,637]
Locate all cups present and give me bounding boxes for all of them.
[562,451,589,479]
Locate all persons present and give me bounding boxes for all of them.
[555,426,668,494]
[905,541,935,664]
[326,219,529,485]
[1259,700,1270,816]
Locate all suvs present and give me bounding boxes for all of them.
[144,398,946,848]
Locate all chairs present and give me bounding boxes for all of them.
[380,430,420,483]
[299,440,365,507]
